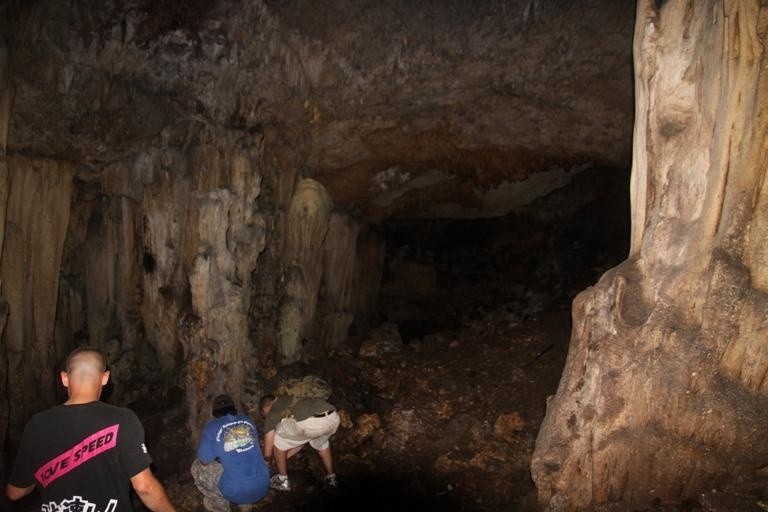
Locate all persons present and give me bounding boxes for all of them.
[259,395,340,493]
[190,394,270,512]
[4,345,176,512]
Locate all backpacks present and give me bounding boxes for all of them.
[272,374,335,404]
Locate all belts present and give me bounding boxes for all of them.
[311,409,337,419]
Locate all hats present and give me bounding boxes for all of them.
[211,393,236,412]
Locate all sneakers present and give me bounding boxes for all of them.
[322,474,339,488]
[268,472,291,491]
[202,497,253,512]
